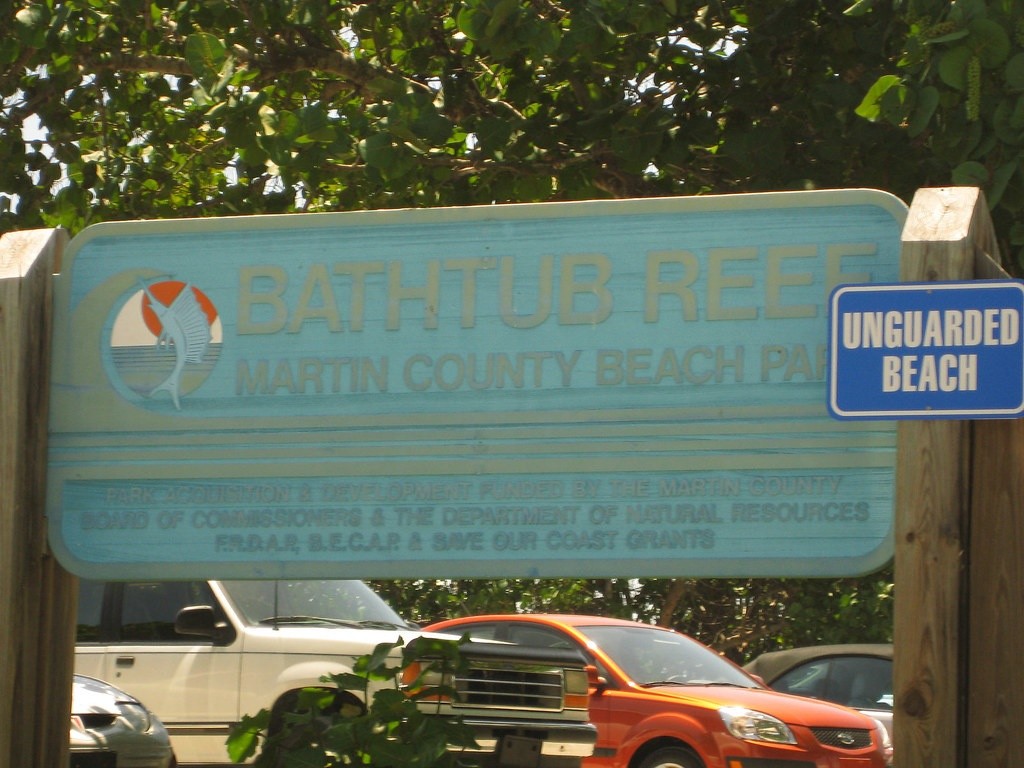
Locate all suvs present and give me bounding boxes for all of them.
[73,573,600,768]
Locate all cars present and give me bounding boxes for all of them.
[735,643,897,750]
[69,672,178,768]
[414,611,895,768]
